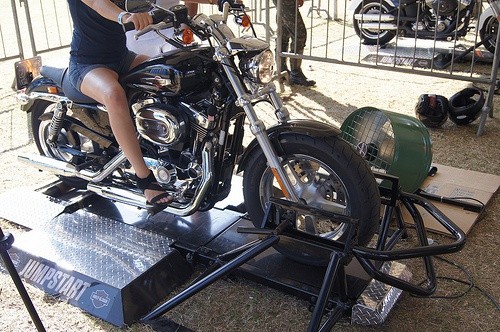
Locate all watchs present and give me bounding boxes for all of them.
[117,11,131,24]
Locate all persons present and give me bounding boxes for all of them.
[272,0,316,87]
[67,0,243,208]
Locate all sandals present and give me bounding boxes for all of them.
[134,170,174,205]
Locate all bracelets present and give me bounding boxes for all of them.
[209,0,214,5]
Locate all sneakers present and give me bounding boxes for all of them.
[290,72,316,86]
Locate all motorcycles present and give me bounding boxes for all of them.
[353,0,500,55]
[11,0,380,268]
